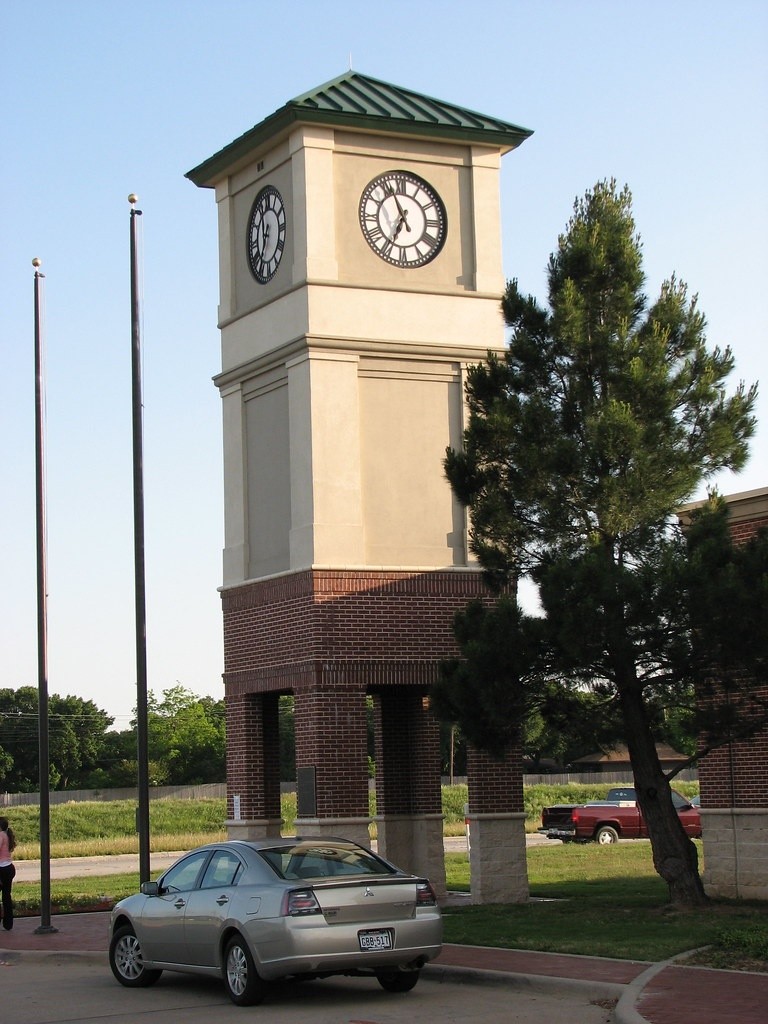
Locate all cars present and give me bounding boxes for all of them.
[109,836,444,1008]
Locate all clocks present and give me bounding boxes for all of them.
[244,186,286,286]
[358,170,448,270]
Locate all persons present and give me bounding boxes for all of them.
[0,817,16,930]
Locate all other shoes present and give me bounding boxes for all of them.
[0,926,12,931]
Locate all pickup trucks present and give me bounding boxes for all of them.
[537,786,703,846]
